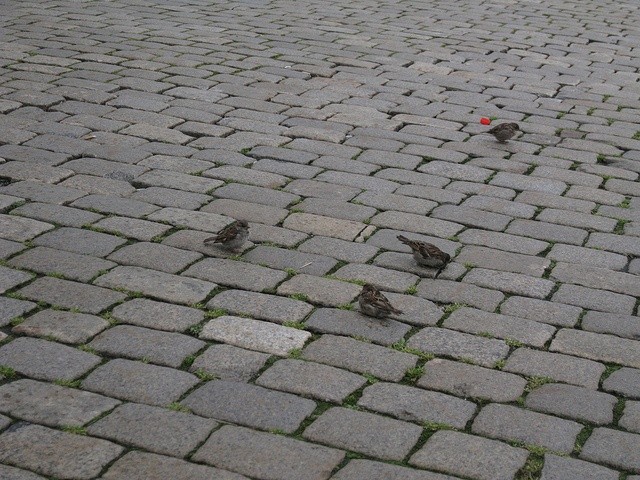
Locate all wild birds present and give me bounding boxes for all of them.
[358,280,403,320]
[396,231,452,270]
[481,121,523,145]
[203,218,251,252]
